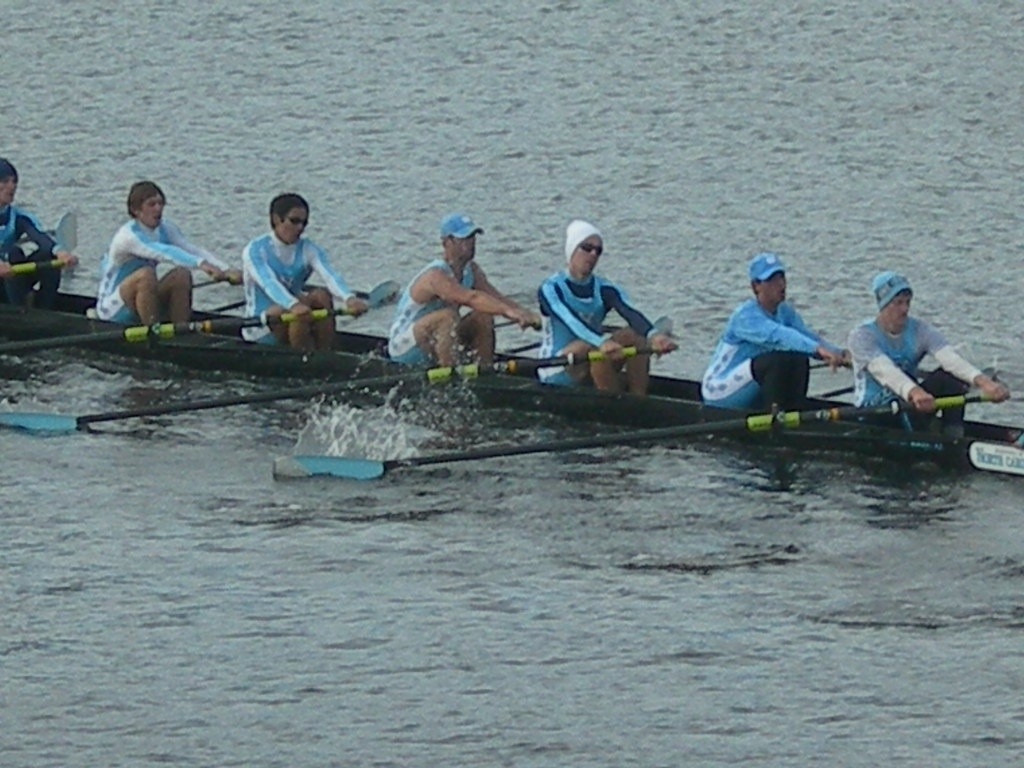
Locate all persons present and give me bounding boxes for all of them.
[242,192,367,350]
[0,157,78,310]
[849,270,1009,436]
[96,180,244,330]
[535,220,679,395]
[701,252,851,413]
[387,214,541,368]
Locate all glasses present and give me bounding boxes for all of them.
[285,215,308,227]
[579,242,603,256]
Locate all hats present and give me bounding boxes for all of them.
[749,253,785,281]
[0,158,18,181]
[565,219,602,263]
[872,271,913,311]
[441,212,485,238]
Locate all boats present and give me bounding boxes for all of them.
[0,288,1024,482]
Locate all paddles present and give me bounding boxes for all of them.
[520,314,679,339]
[272,377,990,481]
[1,341,679,438]
[0,307,360,352]
[211,270,367,298]
[9,256,63,274]
[813,348,1005,376]
